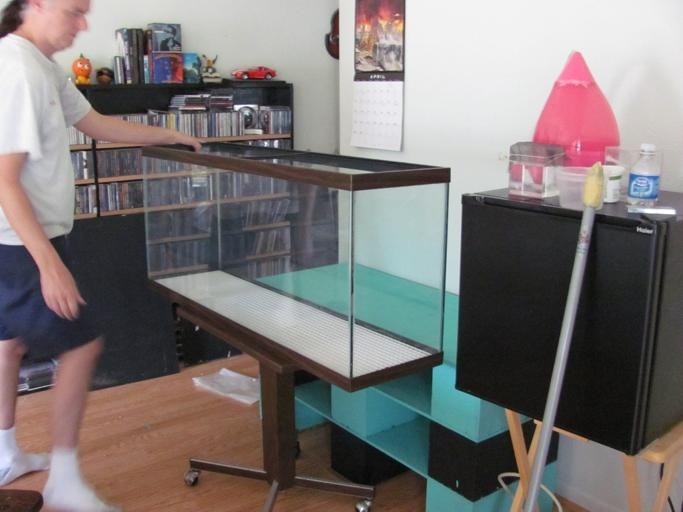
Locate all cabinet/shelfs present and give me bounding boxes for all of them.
[1,80,293,392]
[256,263,557,512]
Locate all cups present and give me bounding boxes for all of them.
[554,164,622,213]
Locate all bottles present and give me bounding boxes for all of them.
[628,145,659,206]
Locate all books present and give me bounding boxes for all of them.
[66,114,144,215]
[114,24,222,85]
[144,94,293,281]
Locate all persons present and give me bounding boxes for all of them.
[0,1,201,511]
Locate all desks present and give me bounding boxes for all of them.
[505,408,683,512]
[144,302,376,512]
